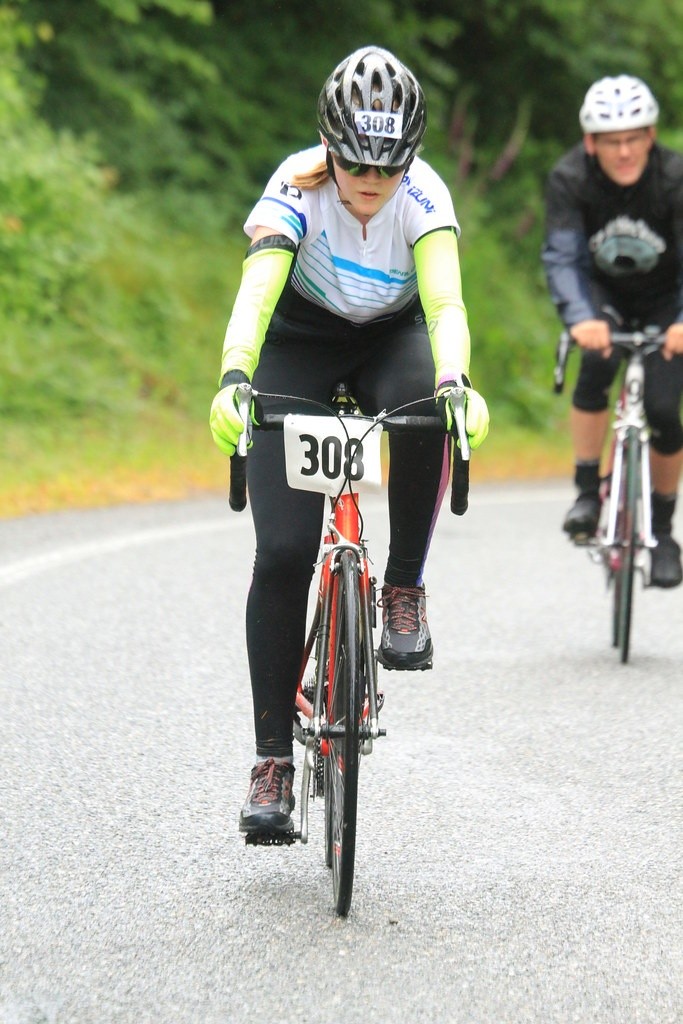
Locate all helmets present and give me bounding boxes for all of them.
[579,74,659,134]
[316,44,427,167]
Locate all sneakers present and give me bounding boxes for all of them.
[239,757,296,832]
[373,581,433,669]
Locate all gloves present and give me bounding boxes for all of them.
[434,372,490,451]
[209,373,264,456]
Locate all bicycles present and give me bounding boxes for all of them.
[553,328,668,663]
[228,382,470,918]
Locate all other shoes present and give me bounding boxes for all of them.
[564,491,603,534]
[650,534,682,587]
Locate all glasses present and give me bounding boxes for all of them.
[332,152,411,179]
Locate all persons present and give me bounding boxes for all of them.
[541,72,683,586]
[205,48,489,830]
